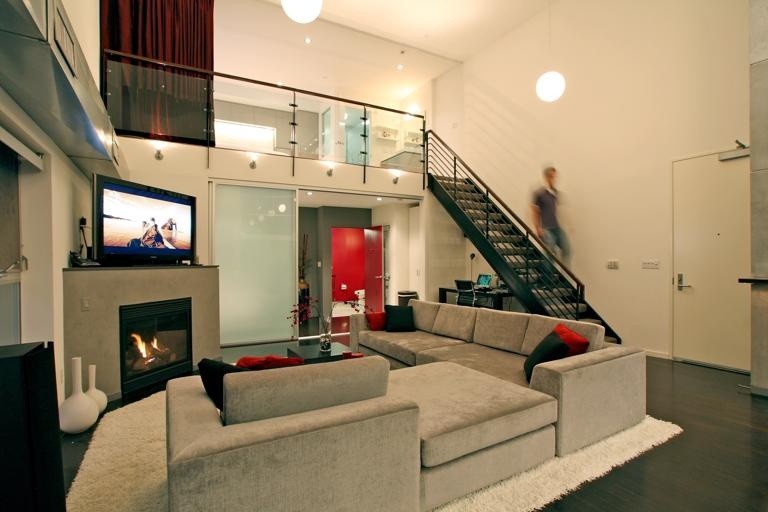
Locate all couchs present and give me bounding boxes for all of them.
[165,354,557,511]
[350,299,645,458]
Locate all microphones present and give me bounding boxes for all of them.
[470,253,477,280]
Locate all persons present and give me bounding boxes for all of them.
[529,166,573,290]
[140,217,165,249]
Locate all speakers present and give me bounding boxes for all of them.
[0,340,66,512]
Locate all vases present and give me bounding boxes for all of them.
[57,356,108,436]
[319,328,331,353]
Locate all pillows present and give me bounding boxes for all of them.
[364,311,384,331]
[196,358,250,413]
[383,304,416,332]
[235,353,305,372]
[523,331,573,385]
[553,323,591,355]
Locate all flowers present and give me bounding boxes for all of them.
[285,300,376,342]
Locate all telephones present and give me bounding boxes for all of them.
[70,250,101,267]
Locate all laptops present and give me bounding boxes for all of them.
[475,274,491,290]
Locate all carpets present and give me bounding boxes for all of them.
[61,369,682,511]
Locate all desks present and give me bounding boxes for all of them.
[439,287,514,310]
[287,341,354,365]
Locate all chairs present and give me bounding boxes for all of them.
[455,279,476,306]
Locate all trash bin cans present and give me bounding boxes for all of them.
[398,291,417,306]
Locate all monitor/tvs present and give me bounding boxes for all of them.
[90,172,197,265]
